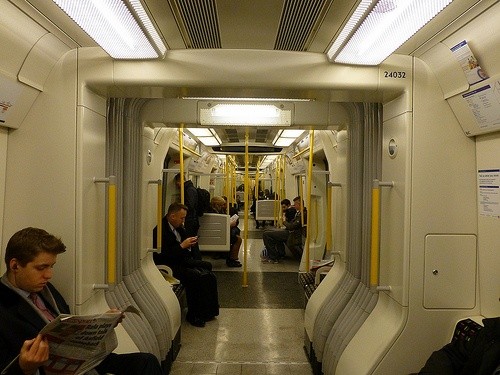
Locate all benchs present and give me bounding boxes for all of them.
[297,266,331,309]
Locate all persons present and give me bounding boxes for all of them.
[152,203,220,328]
[251,181,278,229]
[211,197,242,267]
[238,184,244,191]
[0,227,163,375]
[262,197,306,263]
[175,175,202,262]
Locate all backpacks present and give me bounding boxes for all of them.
[192,186,210,209]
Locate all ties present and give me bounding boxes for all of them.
[29,292,57,324]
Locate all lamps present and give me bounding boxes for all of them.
[52,0,168,61]
[326,0,454,66]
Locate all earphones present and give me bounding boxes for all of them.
[13,264,17,270]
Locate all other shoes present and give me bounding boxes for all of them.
[226,257,242,267]
[261,256,278,264]
